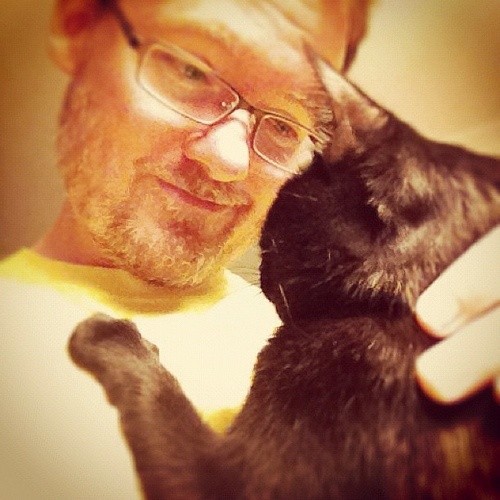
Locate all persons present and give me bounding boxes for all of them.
[0,2,500,500]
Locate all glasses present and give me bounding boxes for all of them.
[109,1,333,177]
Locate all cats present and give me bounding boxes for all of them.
[67,37,499,499]
